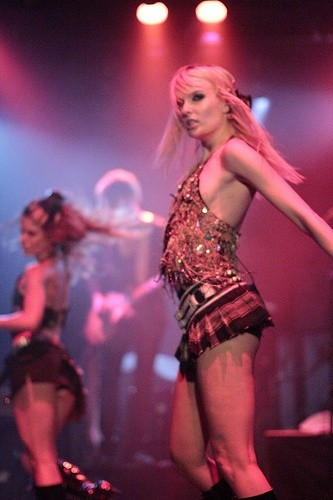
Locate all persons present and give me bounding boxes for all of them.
[75,170,169,474]
[155,63,333,500]
[0,191,132,500]
